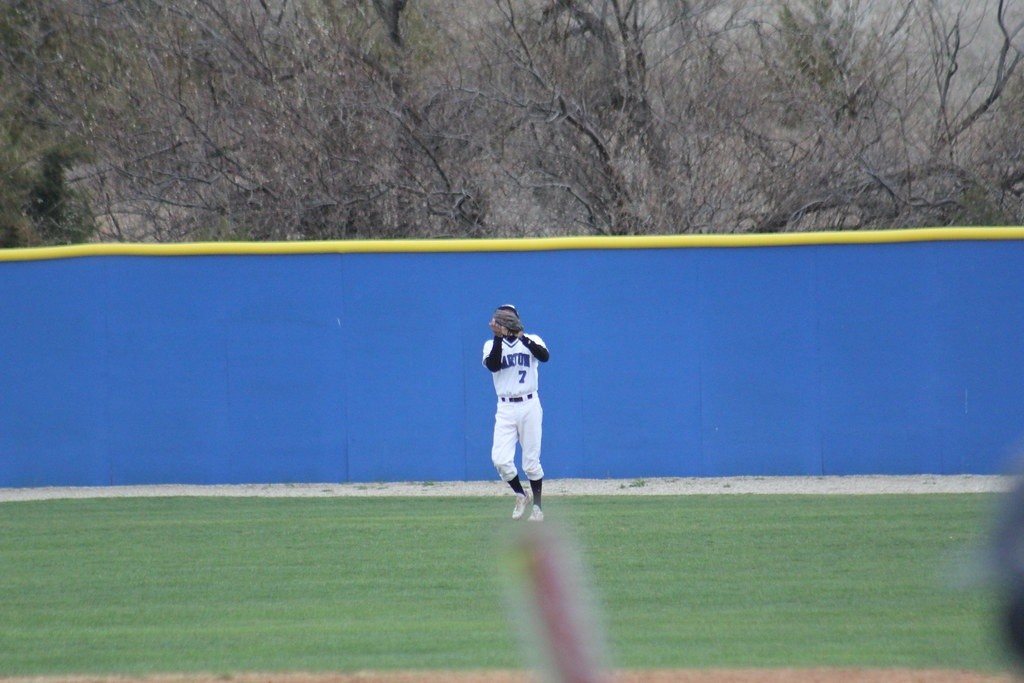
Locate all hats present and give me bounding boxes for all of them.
[498,305,520,319]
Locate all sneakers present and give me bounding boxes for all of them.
[528,505,544,522]
[512,491,531,521]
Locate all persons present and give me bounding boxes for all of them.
[481,304,550,521]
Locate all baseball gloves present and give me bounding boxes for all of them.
[492,308,524,337]
